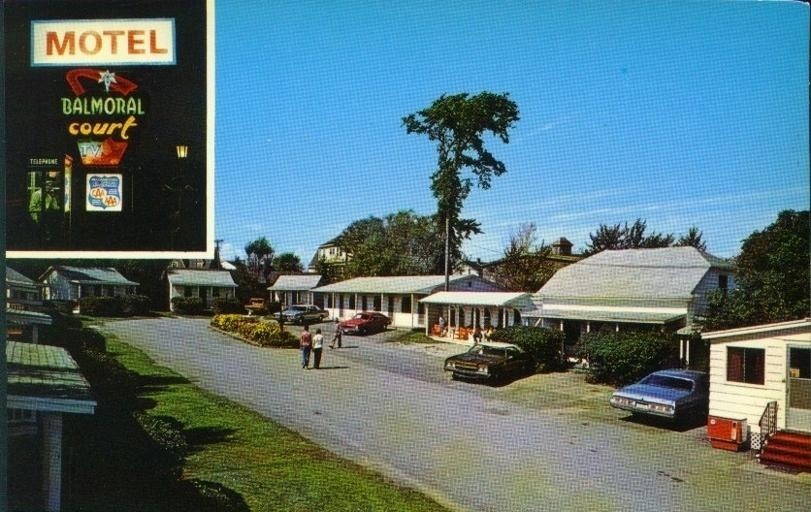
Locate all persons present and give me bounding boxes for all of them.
[439,313,445,337]
[328,317,342,349]
[311,327,323,369]
[299,323,311,370]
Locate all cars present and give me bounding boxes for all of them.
[273,305,329,325]
[609,369,709,419]
[342,313,391,336]
[444,342,525,381]
